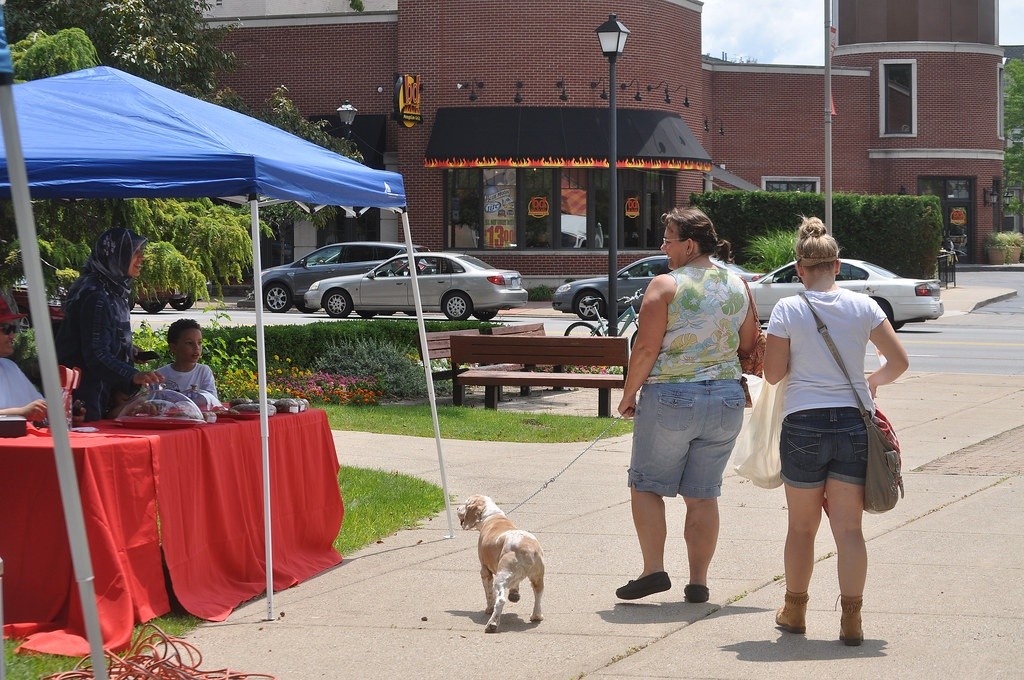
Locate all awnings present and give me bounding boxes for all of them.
[424,107,712,172]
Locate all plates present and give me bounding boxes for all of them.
[113,420,197,430]
[166,411,260,419]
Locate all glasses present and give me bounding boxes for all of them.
[663,237,687,245]
[0,323,16,335]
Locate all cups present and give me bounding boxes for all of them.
[61,386,73,433]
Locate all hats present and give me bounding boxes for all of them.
[0,298,27,321]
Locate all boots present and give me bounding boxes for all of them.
[835,594,865,646]
[776,590,810,634]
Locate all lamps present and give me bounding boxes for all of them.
[514,81,524,103]
[898,185,907,195]
[647,80,671,105]
[462,82,483,102]
[621,78,642,102]
[591,76,609,101]
[983,185,999,207]
[674,84,691,108]
[714,117,726,136]
[558,76,569,103]
[1004,190,1014,208]
[701,112,709,134]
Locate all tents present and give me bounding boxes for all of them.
[0,66,454,620]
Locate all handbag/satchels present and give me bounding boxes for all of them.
[863,408,905,515]
[732,374,788,489]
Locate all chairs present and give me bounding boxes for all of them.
[423,267,432,274]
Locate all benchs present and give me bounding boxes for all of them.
[450,334,630,416]
[419,323,564,406]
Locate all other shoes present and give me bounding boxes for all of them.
[616,571,671,601]
[684,584,710,602]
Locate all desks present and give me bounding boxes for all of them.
[0,407,344,654]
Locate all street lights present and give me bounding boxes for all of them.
[337,98,359,242]
[593,11,631,337]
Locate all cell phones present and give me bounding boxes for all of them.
[73,401,85,416]
[137,351,160,361]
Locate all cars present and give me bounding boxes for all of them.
[304,250,528,321]
[0,236,197,321]
[744,257,945,333]
[551,253,768,321]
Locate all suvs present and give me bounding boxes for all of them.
[252,241,434,314]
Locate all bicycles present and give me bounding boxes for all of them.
[564,288,645,353]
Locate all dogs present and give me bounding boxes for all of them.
[455,495,545,634]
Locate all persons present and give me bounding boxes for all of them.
[0,295,86,423]
[763,217,909,645]
[55,228,164,422]
[616,207,756,602]
[155,319,218,398]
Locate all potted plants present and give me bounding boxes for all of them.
[985,230,1024,265]
